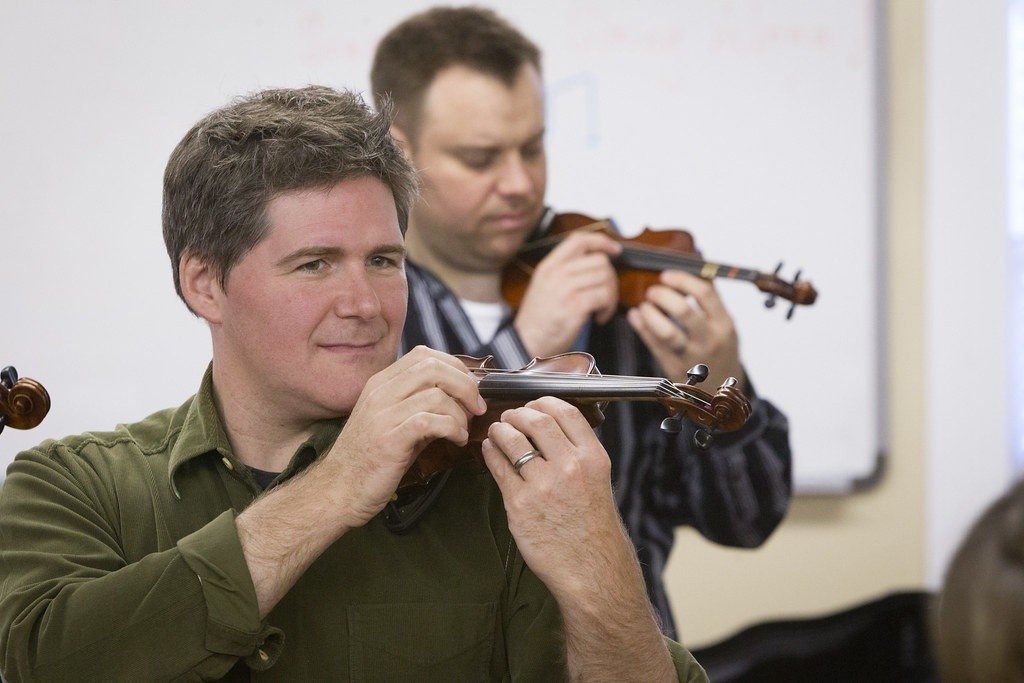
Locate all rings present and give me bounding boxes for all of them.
[512,450,543,474]
[675,342,688,355]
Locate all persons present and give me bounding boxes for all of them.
[1,86,711,683]
[371,6,792,644]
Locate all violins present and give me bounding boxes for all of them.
[0,367,51,432]
[500,205,818,321]
[369,353,753,490]
